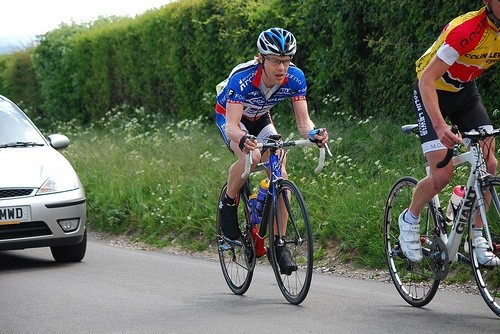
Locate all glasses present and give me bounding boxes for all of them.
[262,54,293,65]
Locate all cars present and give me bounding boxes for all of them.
[0,94,86,263]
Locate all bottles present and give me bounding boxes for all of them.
[254,178,270,211]
[445,184,466,226]
[248,192,260,224]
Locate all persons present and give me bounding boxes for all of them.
[214,27,329,271]
[397,0,500,265]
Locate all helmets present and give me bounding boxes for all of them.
[257,27,297,56]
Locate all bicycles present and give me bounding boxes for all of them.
[216,127,333,305]
[383,123,500,318]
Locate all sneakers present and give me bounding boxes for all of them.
[463,234,500,266]
[218,196,241,240]
[275,245,298,275]
[398,208,423,263]
